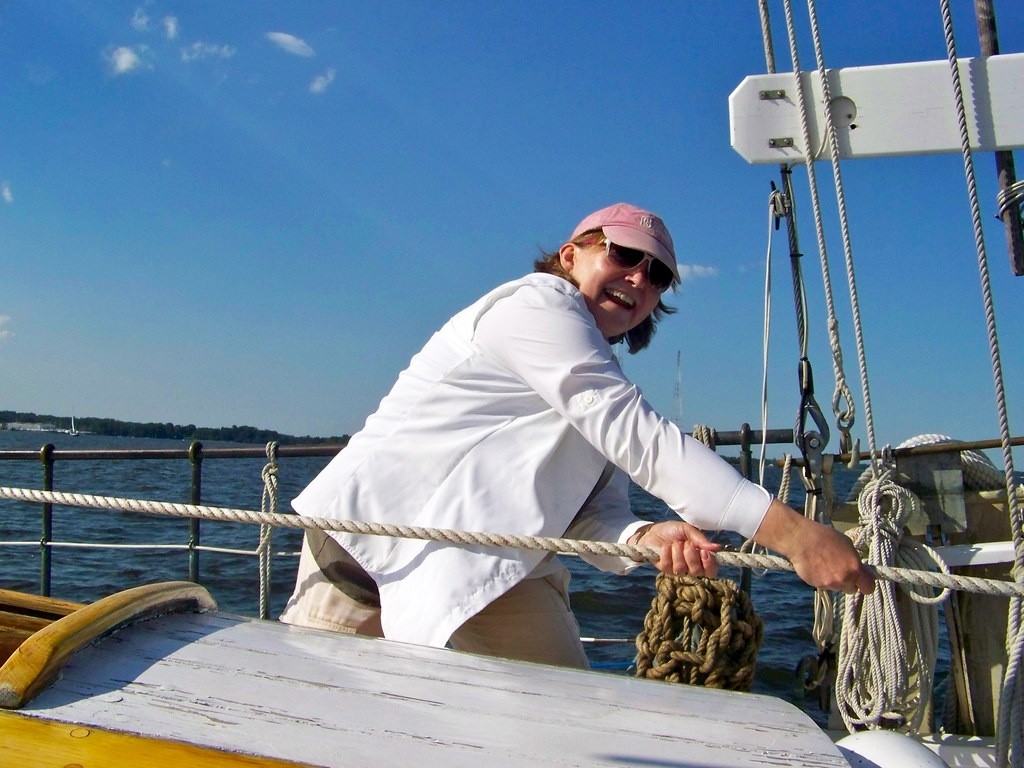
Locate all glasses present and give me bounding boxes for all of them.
[581,236,675,295]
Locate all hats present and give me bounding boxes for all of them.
[567,202,682,286]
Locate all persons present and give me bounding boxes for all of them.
[289,202,876,670]
